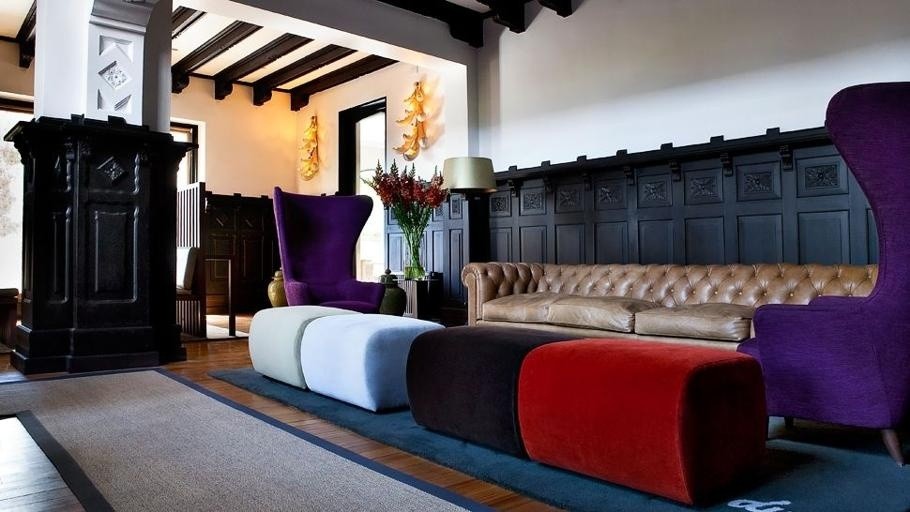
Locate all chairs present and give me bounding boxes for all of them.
[737,82,910,467]
[273,187,385,313]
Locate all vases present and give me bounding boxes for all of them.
[405,267,424,280]
[268,267,287,307]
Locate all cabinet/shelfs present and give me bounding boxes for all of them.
[382,279,442,319]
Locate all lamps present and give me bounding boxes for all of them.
[391,81,428,161]
[300,116,319,181]
[440,157,497,195]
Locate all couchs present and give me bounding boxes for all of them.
[460,262,880,352]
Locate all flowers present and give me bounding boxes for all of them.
[361,159,448,267]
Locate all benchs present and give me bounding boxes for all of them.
[518,338,766,507]
[251,305,356,389]
[301,313,447,412]
[177,246,206,337]
[406,326,580,459]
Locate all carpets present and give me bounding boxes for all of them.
[178,324,248,343]
[0,367,491,512]
[208,369,910,512]
